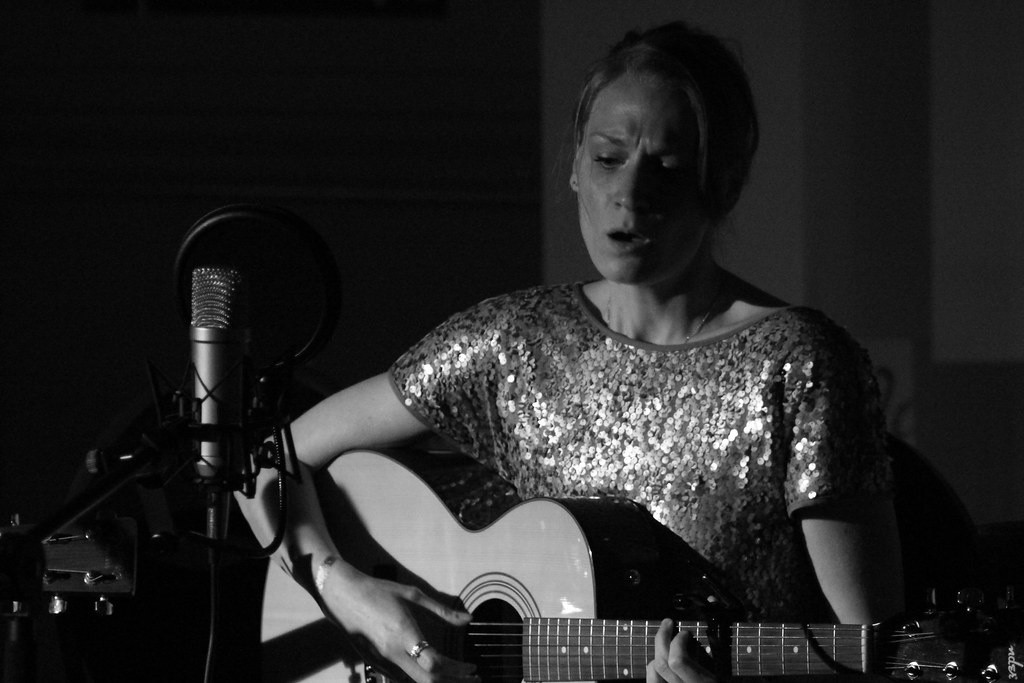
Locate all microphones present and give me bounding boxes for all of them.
[188,266,244,483]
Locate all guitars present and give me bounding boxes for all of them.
[260,443,1024,683]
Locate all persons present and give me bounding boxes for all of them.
[230,22,910,682]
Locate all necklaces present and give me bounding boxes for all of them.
[607,267,725,344]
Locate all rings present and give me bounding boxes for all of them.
[410,640,431,664]
